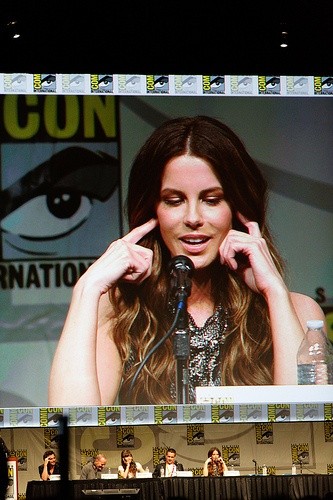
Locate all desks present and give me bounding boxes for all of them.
[26,474,333,500]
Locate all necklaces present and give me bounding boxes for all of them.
[185,302,228,373]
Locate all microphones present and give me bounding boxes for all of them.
[167,255,195,309]
[214,460,218,466]
[253,459,256,463]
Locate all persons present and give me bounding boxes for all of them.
[38,451,60,481]
[80,454,108,480]
[117,449,144,478]
[201,447,227,477]
[152,449,183,478]
[47,116,326,407]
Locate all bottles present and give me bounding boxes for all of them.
[231,465,234,472]
[263,466,267,475]
[160,466,164,477]
[108,468,111,474]
[292,464,296,475]
[297,320,333,385]
[145,466,149,473]
